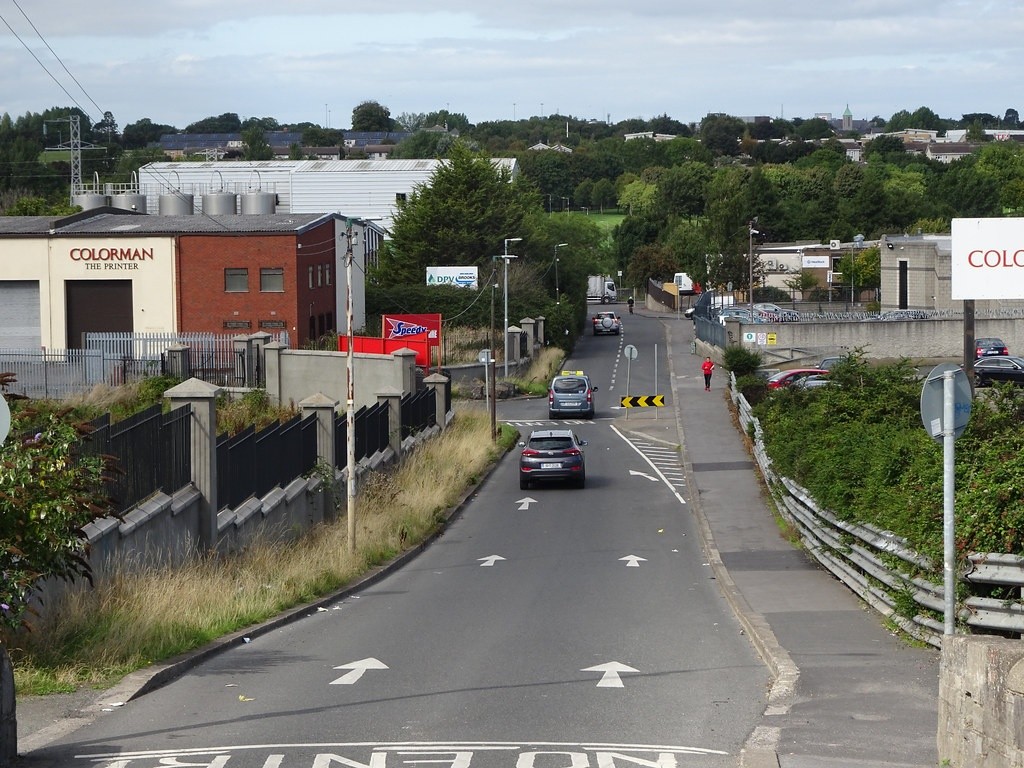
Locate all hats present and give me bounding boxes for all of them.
[629,297,632,299]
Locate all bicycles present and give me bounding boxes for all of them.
[628,304,634,314]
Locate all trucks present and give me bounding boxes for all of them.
[585,275,618,305]
[684,297,735,321]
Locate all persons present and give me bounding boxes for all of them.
[701,357,714,391]
[627,297,634,314]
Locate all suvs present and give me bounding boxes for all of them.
[516,428,589,490]
[592,311,621,336]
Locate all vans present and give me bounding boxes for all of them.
[548,370,599,420]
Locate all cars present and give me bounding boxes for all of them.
[974,337,1009,359]
[960,355,1024,387]
[752,303,799,322]
[717,306,779,326]
[766,369,831,391]
[816,356,846,369]
[858,309,930,320]
[791,373,843,389]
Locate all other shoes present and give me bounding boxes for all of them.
[708,387,711,391]
[705,386,707,390]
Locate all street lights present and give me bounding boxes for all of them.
[546,193,553,219]
[488,254,520,444]
[555,240,569,304]
[560,196,571,216]
[342,211,385,555]
[503,236,524,377]
